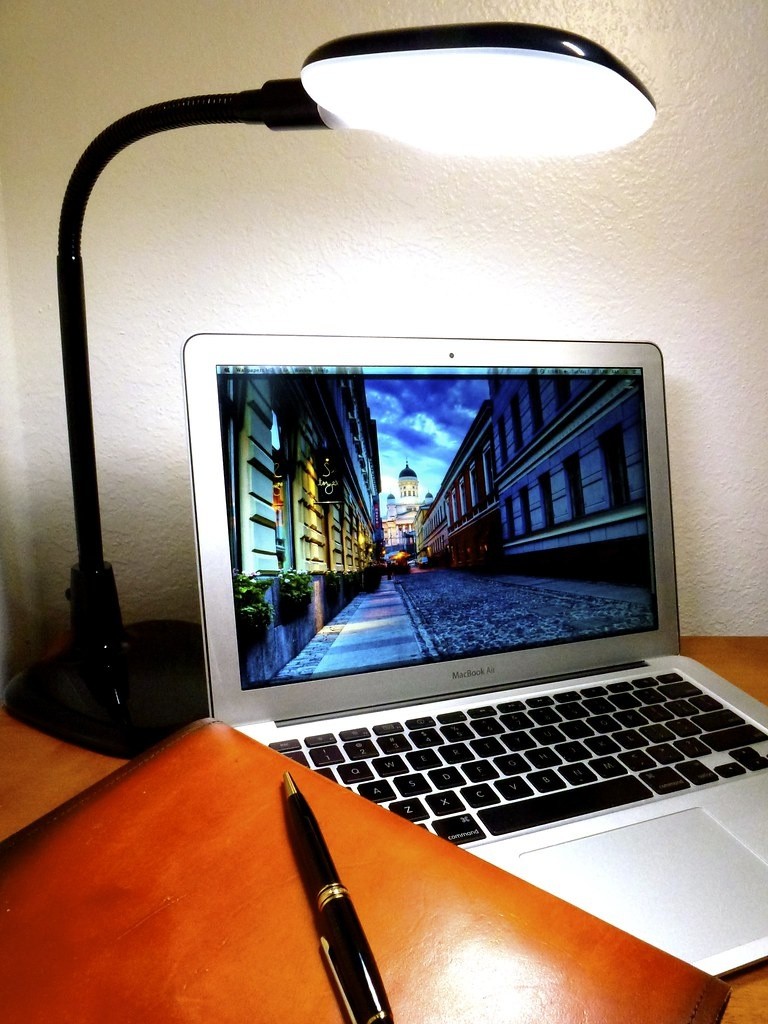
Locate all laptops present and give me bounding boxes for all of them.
[180,333,768,980]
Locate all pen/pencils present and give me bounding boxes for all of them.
[284,774,395,1024]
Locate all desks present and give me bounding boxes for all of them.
[0,637,768,1024]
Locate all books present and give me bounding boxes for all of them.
[0,717,731,1024]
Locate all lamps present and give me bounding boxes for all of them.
[0,14,669,760]
